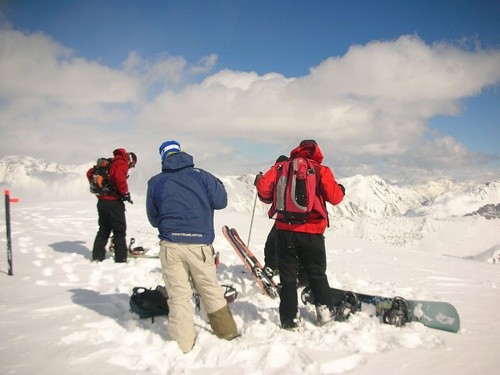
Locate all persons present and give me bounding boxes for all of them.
[87,148,137,263]
[254,140,345,332]
[146,141,238,353]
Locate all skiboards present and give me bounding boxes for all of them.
[222,225,279,300]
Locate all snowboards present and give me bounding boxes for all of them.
[301,286,460,334]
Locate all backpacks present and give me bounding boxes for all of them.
[271,158,323,216]
[129,286,168,318]
[91,157,125,194]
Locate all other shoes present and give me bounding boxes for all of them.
[315,302,336,322]
[263,265,280,287]
[280,317,299,331]
[295,271,308,288]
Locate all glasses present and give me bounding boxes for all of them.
[129,162,134,168]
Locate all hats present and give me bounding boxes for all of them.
[275,155,290,163]
[159,140,180,161]
[130,152,137,164]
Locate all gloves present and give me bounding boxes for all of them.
[338,184,346,195]
[120,192,133,203]
[254,173,263,185]
